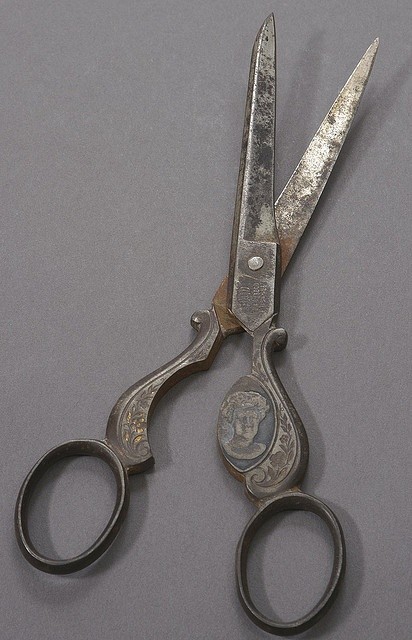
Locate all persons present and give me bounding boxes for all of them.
[220,391,270,460]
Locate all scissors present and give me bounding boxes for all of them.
[14,11,381,636]
